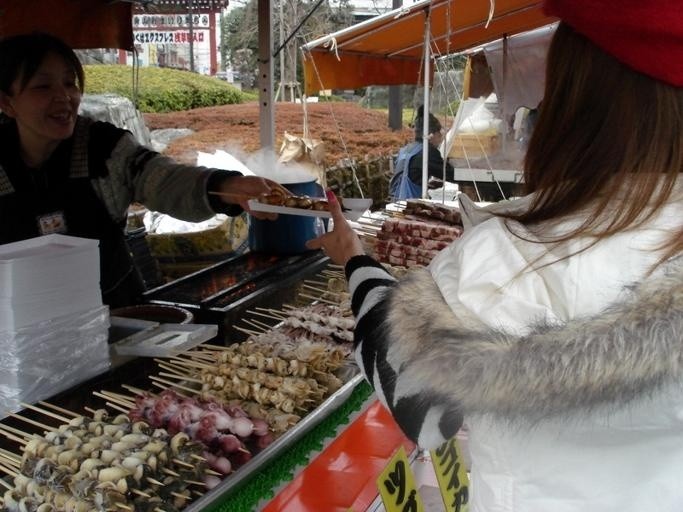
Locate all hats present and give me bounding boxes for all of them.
[543,1,683,89]
[413,104,441,139]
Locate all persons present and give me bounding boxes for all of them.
[3,28,293,303]
[300,0,682,511]
[384,105,464,203]
